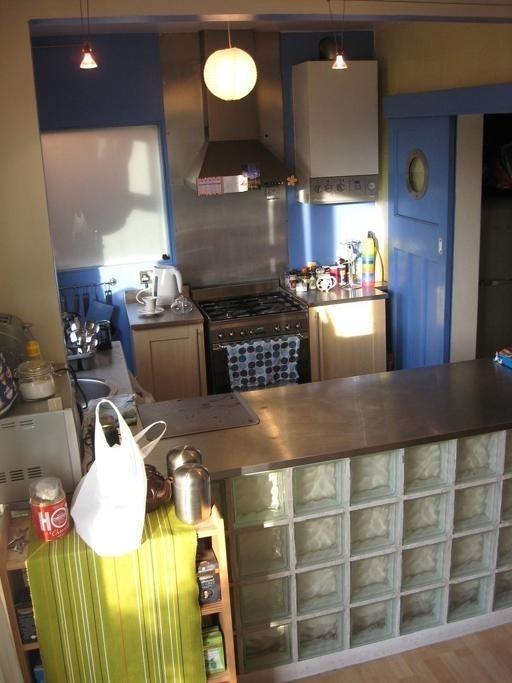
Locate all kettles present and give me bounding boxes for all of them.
[151,266,184,308]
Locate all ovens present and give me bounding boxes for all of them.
[209,331,312,395]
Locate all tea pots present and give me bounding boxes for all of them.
[145,464,175,514]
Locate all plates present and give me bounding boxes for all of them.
[137,307,164,316]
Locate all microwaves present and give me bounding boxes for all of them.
[0,363,85,504]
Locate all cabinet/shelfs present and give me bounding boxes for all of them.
[309,298,386,383]
[133,324,208,402]
[0,504,237,683]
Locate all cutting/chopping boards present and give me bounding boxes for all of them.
[137,390,260,441]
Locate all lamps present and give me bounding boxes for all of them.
[203,21,258,101]
[327,0,348,70]
[79,1,98,69]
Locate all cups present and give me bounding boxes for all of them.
[316,273,337,291]
[142,296,158,313]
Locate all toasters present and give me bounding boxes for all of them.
[0,313,37,368]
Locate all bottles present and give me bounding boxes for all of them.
[282,261,351,292]
[16,359,56,403]
[362,238,375,287]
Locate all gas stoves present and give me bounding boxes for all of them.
[197,290,309,343]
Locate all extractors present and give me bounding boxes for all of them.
[184,29,299,196]
